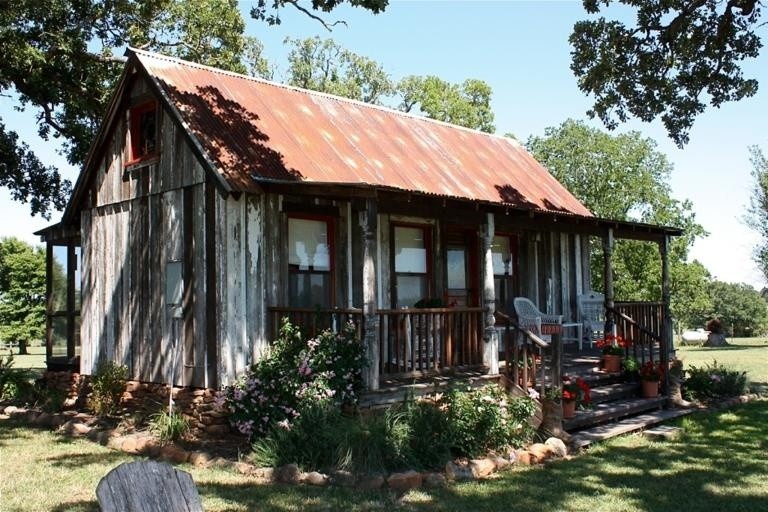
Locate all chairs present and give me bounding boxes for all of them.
[513,296,565,363]
[575,291,618,349]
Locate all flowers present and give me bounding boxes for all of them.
[506,343,544,372]
[639,360,667,383]
[559,371,593,403]
[594,332,636,356]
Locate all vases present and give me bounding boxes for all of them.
[517,366,537,387]
[640,381,660,398]
[561,399,576,419]
[603,354,621,373]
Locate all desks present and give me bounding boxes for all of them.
[494,322,584,361]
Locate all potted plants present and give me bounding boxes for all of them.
[621,358,640,384]
